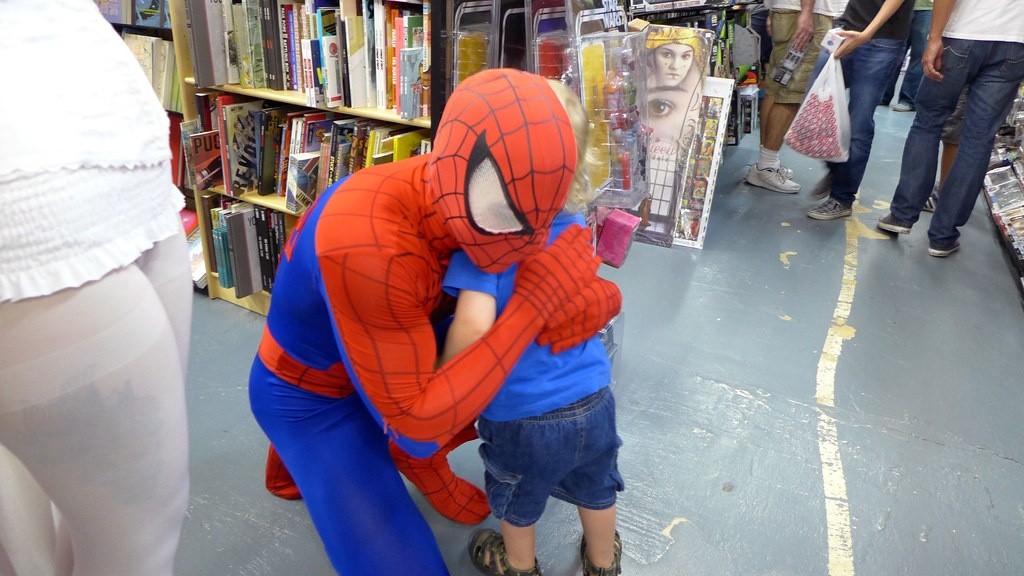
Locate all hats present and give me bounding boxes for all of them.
[644,29,699,61]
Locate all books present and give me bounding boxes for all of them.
[92,0,430,299]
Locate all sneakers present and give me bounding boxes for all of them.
[779,165,795,181]
[806,198,852,220]
[745,163,801,193]
[809,171,833,201]
[927,240,959,257]
[877,212,911,234]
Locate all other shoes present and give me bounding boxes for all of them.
[893,100,912,111]
[922,185,940,211]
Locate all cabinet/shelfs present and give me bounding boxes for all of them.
[168,0,526,320]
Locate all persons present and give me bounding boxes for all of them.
[246,68,623,576]
[644,25,713,147]
[0,0,193,576]
[746,0,1024,258]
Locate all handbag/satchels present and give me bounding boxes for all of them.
[783,50,851,162]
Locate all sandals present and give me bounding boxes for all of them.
[581,527,622,576]
[469,528,543,576]
